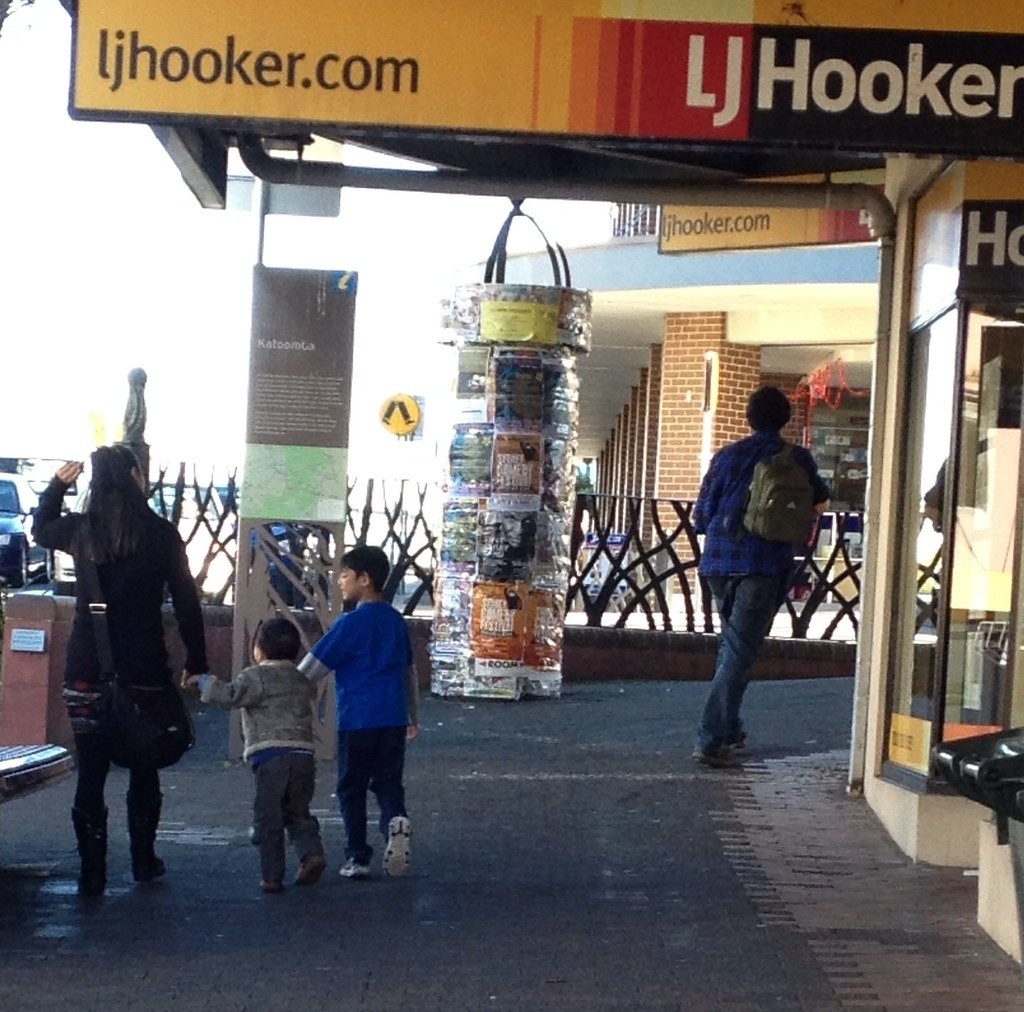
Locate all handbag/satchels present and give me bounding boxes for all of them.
[98,673,195,767]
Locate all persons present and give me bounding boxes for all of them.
[189,619,326,893]
[31,445,209,897]
[298,546,418,879]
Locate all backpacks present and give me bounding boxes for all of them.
[730,441,815,543]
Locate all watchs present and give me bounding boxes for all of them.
[693,386,831,773]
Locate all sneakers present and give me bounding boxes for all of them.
[339,859,369,877]
[383,815,412,879]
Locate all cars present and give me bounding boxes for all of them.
[0,473,55,588]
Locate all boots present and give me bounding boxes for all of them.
[71,807,107,898]
[126,791,166,879]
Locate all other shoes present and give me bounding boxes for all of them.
[294,855,326,887]
[259,880,281,892]
[692,746,729,765]
